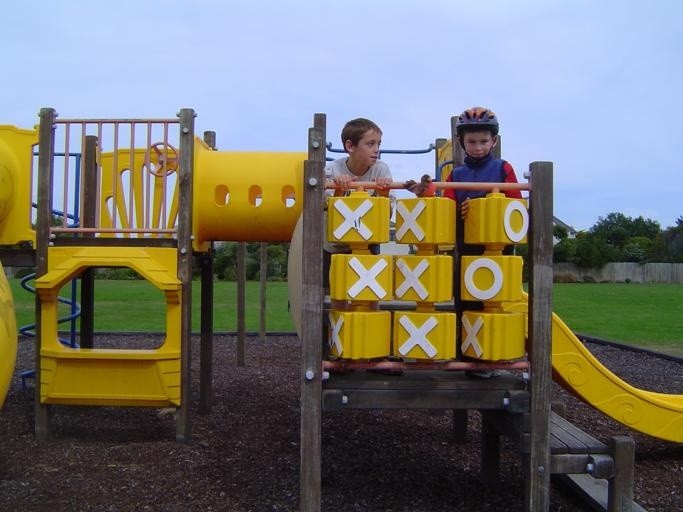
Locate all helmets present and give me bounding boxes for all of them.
[456,107,499,135]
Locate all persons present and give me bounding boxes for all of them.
[322,116,404,364]
[441,106,522,364]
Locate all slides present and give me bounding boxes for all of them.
[523,292,683,444]
